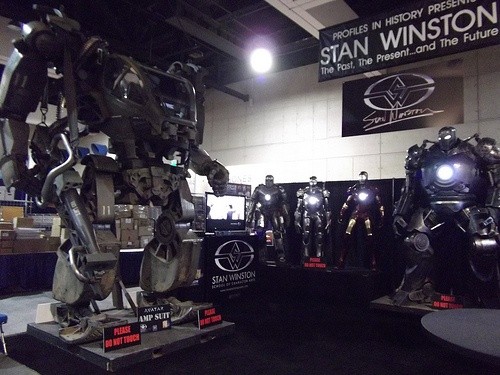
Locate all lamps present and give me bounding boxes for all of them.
[249,47,274,75]
[23,23,33,35]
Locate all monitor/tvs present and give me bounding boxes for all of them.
[204,192,246,232]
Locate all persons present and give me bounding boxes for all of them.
[389,124,499,310]
[226,204,236,219]
[292,175,332,266]
[0,0,231,348]
[247,174,292,268]
[336,170,386,272]
[206,205,212,220]
